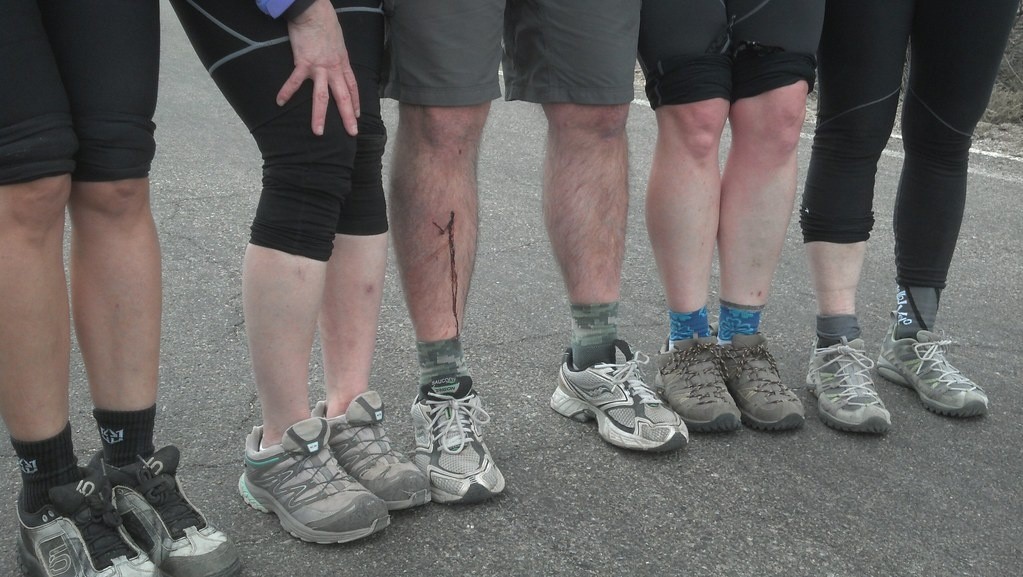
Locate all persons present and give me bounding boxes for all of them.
[637,0,828,438]
[165,0,434,550]
[806,2,1023,440]
[0,0,239,577]
[376,0,700,510]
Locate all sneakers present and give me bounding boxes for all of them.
[551,337,690,452]
[16,465,162,577]
[236,416,392,544]
[409,374,505,506]
[86,444,242,577]
[311,389,432,511]
[709,321,807,431]
[876,310,989,417]
[651,330,742,433]
[805,332,892,434]
